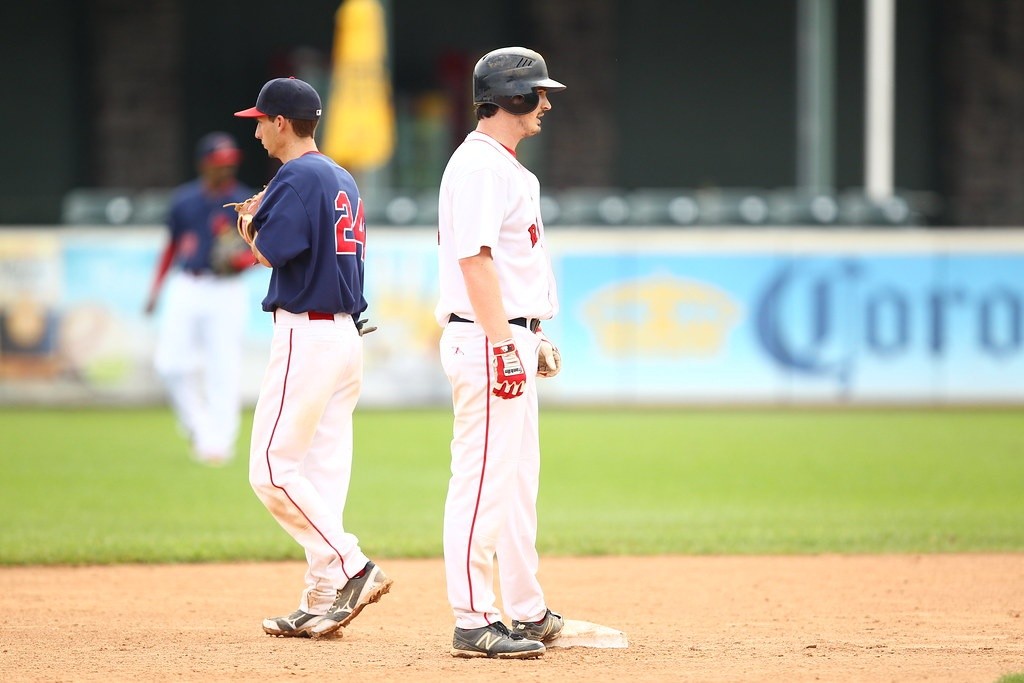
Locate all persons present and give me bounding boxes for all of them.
[223,76,391,638]
[437,47,567,658]
[143,133,258,462]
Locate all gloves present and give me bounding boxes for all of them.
[492,338,526,399]
[357,319,377,337]
[535,327,561,378]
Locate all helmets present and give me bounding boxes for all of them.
[473,46,567,115]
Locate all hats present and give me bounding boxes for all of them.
[234,76,322,120]
[197,135,239,169]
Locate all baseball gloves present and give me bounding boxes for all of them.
[222,184,269,245]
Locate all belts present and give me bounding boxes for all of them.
[448,312,541,333]
[306,310,335,321]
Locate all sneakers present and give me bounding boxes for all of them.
[514,608,565,643]
[262,608,342,637]
[310,561,393,636]
[449,621,546,660]
